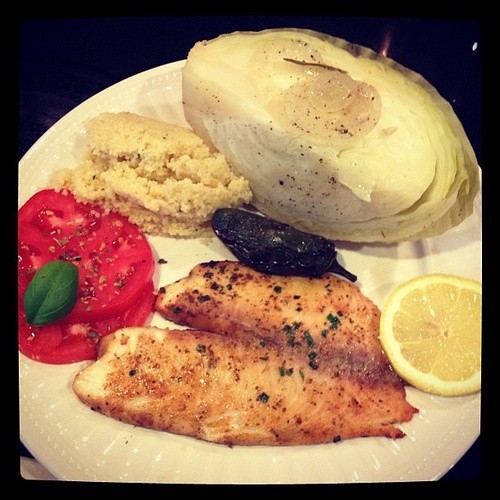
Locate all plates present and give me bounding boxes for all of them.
[17,58,480,483]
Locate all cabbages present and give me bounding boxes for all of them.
[180,27,482,246]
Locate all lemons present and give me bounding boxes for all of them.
[378,273,482,398]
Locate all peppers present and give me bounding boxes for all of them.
[210,208,360,285]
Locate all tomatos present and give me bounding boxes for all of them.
[18,188,157,366]
